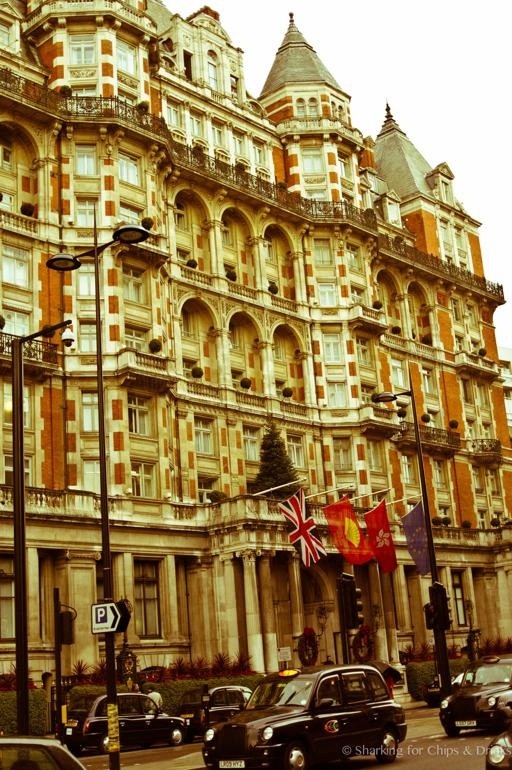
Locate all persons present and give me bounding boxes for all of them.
[322,655,336,666]
[383,670,395,700]
[128,682,143,713]
[144,685,163,714]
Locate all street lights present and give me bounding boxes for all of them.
[46,202,152,770]
[374,366,452,703]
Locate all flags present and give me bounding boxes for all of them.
[276,486,328,570]
[363,495,399,574]
[321,493,375,567]
[399,498,434,576]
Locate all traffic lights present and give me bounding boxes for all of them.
[433,581,453,630]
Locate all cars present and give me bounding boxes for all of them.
[423,654,512,770]
[0,692,255,770]
[202,663,408,769]
